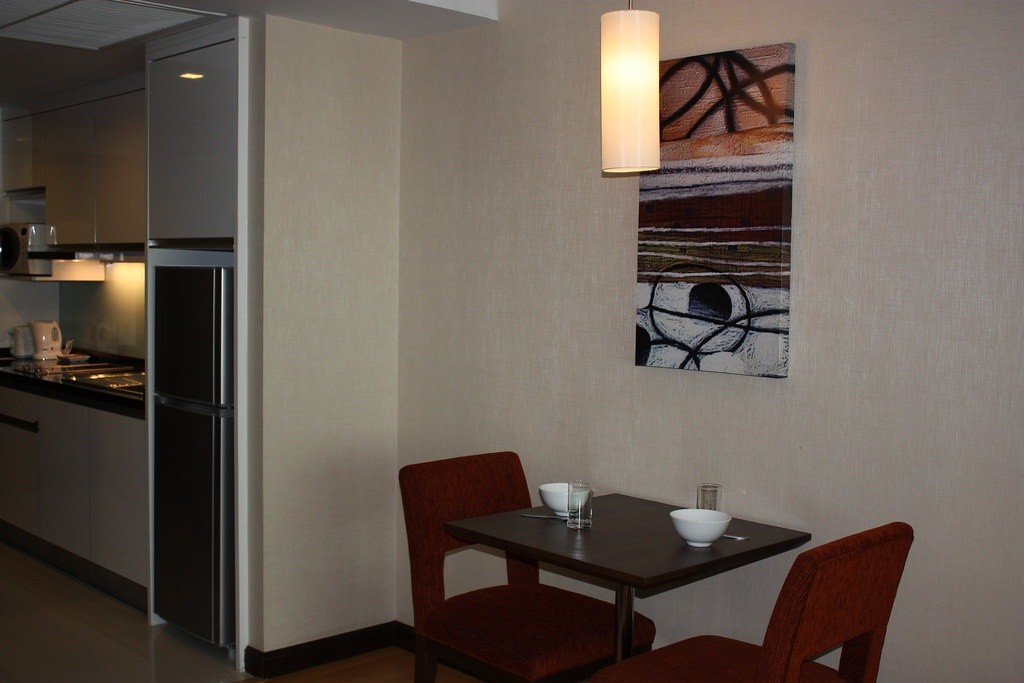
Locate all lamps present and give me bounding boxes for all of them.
[600,0,661,173]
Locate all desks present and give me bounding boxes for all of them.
[444,492,812,662]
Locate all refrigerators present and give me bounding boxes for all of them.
[153,265,236,659]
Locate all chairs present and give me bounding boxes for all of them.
[591,521,914,683]
[398,451,656,683]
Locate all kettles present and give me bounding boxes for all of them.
[28,319,62,360]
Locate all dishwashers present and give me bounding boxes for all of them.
[0,386,41,539]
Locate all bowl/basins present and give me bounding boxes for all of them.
[668,509,732,548]
[538,482,589,517]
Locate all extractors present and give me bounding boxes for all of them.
[27,250,145,267]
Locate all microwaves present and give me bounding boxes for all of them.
[0,222,75,277]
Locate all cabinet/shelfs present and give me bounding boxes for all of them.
[44,89,146,244]
[39,396,148,588]
[0,387,39,538]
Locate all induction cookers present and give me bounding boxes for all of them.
[12,363,134,378]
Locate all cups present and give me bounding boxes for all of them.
[696,483,722,512]
[566,479,592,530]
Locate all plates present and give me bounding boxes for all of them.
[56,354,90,363]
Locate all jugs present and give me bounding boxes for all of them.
[7,325,36,358]
